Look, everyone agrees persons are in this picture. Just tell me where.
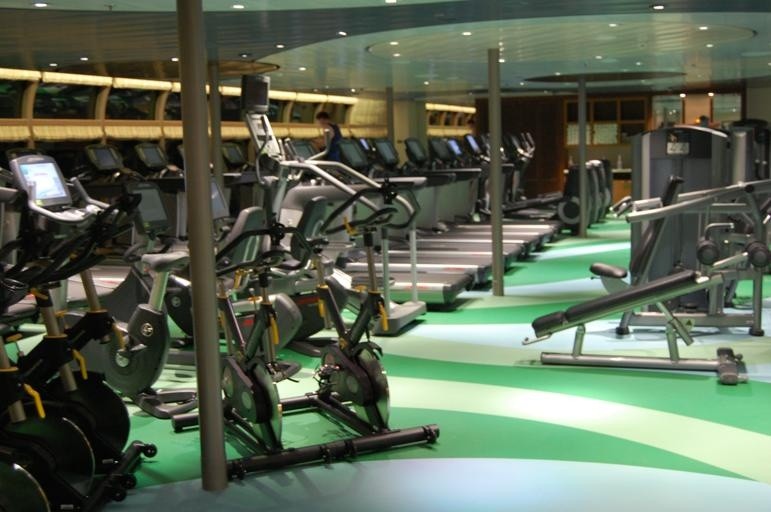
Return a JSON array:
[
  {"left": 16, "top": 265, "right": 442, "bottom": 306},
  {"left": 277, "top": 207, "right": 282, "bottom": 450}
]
[
  {"left": 310, "top": 110, "right": 342, "bottom": 161},
  {"left": 696, "top": 115, "right": 709, "bottom": 128}
]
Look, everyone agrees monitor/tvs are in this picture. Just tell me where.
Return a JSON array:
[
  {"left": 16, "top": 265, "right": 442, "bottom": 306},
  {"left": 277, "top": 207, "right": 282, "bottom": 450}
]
[
  {"left": 288, "top": 134, "right": 482, "bottom": 170},
  {"left": 9, "top": 154, "right": 73, "bottom": 207},
  {"left": 241, "top": 74, "right": 272, "bottom": 113},
  {"left": 221, "top": 142, "right": 247, "bottom": 164},
  {"left": 83, "top": 142, "right": 125, "bottom": 170},
  {"left": 134, "top": 142, "right": 170, "bottom": 168}
]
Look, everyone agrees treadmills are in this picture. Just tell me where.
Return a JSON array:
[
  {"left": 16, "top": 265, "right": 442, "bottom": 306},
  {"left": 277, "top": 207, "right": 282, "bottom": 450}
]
[
  {"left": 351, "top": 135, "right": 526, "bottom": 271},
  {"left": 318, "top": 134, "right": 508, "bottom": 288},
  {"left": 400, "top": 133, "right": 559, "bottom": 271},
  {"left": 284, "top": 137, "right": 476, "bottom": 307}
]
